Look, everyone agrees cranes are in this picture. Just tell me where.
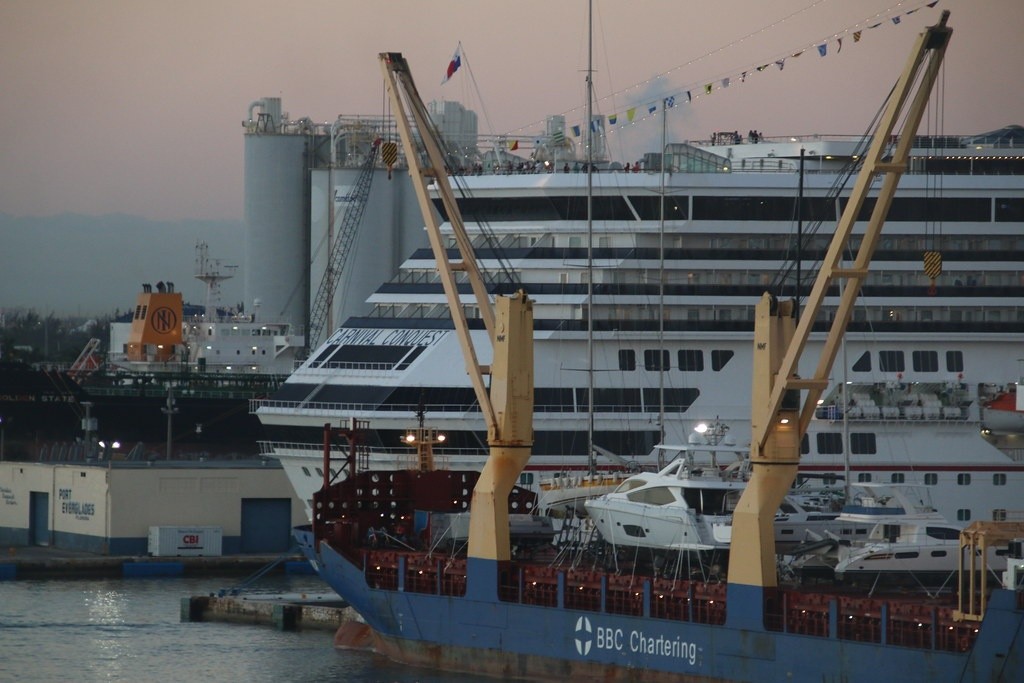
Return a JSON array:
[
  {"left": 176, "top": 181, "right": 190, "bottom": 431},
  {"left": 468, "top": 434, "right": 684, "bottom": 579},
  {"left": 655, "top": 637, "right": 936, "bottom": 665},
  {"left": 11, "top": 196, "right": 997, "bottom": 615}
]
[
  {"left": 724, "top": 10, "right": 955, "bottom": 630},
  {"left": 380, "top": 51, "right": 528, "bottom": 600}
]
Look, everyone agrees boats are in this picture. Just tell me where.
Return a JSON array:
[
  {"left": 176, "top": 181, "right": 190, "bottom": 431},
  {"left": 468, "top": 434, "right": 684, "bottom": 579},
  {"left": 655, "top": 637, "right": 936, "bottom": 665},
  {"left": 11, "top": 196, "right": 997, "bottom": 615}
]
[{"left": 290, "top": 399, "right": 1024, "bottom": 683}]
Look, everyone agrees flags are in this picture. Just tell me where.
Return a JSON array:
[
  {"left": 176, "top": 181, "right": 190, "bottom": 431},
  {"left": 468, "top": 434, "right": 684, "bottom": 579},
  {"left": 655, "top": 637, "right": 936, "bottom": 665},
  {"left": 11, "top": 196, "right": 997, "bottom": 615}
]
[
  {"left": 441, "top": 45, "right": 461, "bottom": 86},
  {"left": 742, "top": 72, "right": 747, "bottom": 81},
  {"left": 705, "top": 84, "right": 712, "bottom": 95},
  {"left": 533, "top": 136, "right": 542, "bottom": 150},
  {"left": 554, "top": 131, "right": 565, "bottom": 145},
  {"left": 509, "top": 140, "right": 520, "bottom": 150},
  {"left": 591, "top": 119, "right": 600, "bottom": 133},
  {"left": 757, "top": 0, "right": 938, "bottom": 72},
  {"left": 722, "top": 79, "right": 729, "bottom": 88},
  {"left": 648, "top": 103, "right": 657, "bottom": 115},
  {"left": 609, "top": 114, "right": 617, "bottom": 125},
  {"left": 627, "top": 107, "right": 634, "bottom": 122},
  {"left": 570, "top": 125, "right": 580, "bottom": 137},
  {"left": 666, "top": 97, "right": 675, "bottom": 109}
]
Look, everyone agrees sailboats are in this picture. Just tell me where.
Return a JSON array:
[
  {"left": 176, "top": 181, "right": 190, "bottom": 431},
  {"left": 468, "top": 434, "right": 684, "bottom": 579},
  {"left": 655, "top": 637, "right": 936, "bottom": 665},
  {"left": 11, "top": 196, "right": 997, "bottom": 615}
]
[{"left": 538, "top": 0, "right": 1009, "bottom": 590}]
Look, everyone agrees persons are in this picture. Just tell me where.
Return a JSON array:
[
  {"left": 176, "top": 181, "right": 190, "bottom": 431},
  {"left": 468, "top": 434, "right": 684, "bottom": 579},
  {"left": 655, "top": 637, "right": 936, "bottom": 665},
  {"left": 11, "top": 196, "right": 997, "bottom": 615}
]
[
  {"left": 445, "top": 159, "right": 639, "bottom": 176},
  {"left": 712, "top": 130, "right": 764, "bottom": 145}
]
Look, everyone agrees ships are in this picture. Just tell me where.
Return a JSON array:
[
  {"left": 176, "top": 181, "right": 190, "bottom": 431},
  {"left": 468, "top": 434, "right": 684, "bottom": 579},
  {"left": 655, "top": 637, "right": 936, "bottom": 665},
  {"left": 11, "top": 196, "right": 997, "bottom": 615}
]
[{"left": 250, "top": 97, "right": 1024, "bottom": 538}]
